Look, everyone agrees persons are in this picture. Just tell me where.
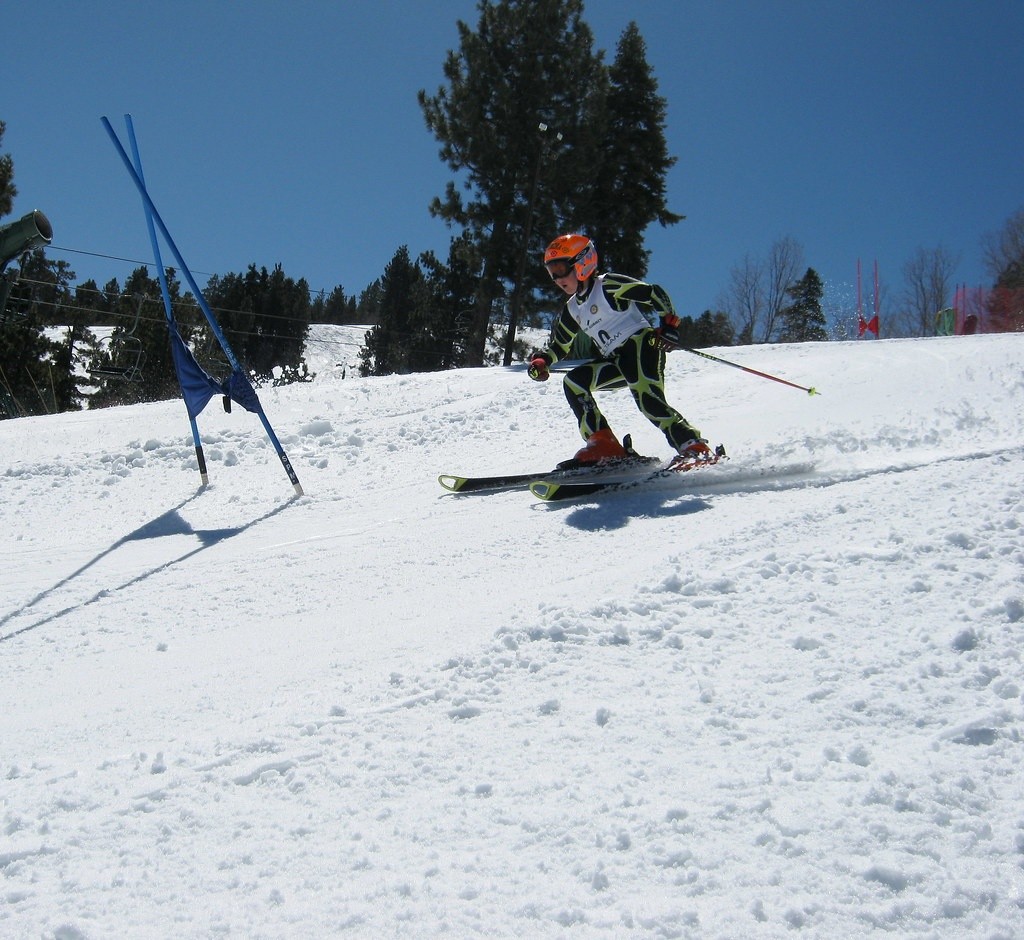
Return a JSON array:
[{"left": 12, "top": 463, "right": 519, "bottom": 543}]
[{"left": 527, "top": 234, "right": 731, "bottom": 482}]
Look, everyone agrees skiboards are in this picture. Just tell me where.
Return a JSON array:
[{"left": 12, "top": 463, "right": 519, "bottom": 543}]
[{"left": 438, "top": 433, "right": 731, "bottom": 503}]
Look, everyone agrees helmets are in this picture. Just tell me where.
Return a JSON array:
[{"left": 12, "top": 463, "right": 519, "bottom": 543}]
[{"left": 543, "top": 234, "right": 598, "bottom": 281}]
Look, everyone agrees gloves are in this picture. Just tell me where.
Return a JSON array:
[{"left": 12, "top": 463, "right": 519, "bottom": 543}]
[
  {"left": 648, "top": 315, "right": 681, "bottom": 353},
  {"left": 528, "top": 352, "right": 553, "bottom": 381}
]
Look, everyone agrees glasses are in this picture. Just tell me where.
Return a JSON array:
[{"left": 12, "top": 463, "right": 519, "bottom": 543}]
[{"left": 543, "top": 256, "right": 574, "bottom": 279}]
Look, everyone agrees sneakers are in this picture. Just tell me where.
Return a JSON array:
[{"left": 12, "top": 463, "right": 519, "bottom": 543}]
[
  {"left": 668, "top": 439, "right": 717, "bottom": 472},
  {"left": 574, "top": 429, "right": 624, "bottom": 461}
]
